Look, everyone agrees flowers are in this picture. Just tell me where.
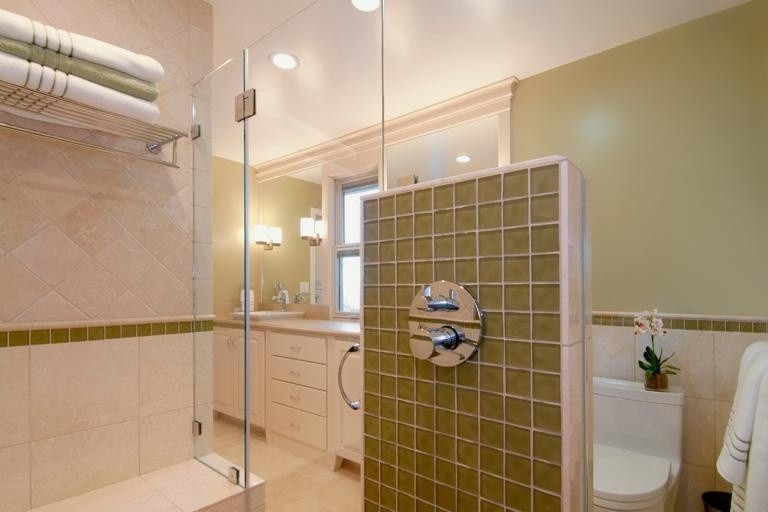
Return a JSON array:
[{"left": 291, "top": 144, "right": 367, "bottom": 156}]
[{"left": 633, "top": 308, "right": 680, "bottom": 380}]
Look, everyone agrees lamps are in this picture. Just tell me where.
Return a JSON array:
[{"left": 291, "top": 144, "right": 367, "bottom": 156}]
[
  {"left": 315, "top": 220, "right": 322, "bottom": 246},
  {"left": 269, "top": 227, "right": 281, "bottom": 250},
  {"left": 255, "top": 226, "right": 269, "bottom": 250},
  {"left": 300, "top": 217, "right": 315, "bottom": 246}
]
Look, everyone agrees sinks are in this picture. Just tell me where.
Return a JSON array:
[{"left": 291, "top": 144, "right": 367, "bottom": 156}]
[{"left": 252, "top": 308, "right": 307, "bottom": 320}]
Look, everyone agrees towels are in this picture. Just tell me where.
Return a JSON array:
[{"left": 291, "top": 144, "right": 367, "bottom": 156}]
[{"left": 0, "top": 9, "right": 162, "bottom": 125}]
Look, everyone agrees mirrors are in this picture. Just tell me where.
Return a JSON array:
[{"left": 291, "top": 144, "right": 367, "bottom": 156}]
[{"left": 258, "top": 162, "right": 323, "bottom": 306}]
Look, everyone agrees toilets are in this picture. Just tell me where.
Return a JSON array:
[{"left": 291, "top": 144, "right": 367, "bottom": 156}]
[{"left": 590, "top": 376, "right": 687, "bottom": 512}]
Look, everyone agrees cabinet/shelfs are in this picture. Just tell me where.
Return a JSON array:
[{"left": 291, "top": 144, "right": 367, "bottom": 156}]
[{"left": 206, "top": 326, "right": 362, "bottom": 479}]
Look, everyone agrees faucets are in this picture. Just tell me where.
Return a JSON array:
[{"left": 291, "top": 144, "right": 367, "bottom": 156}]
[
  {"left": 294, "top": 290, "right": 306, "bottom": 303},
  {"left": 271, "top": 280, "right": 289, "bottom": 312}
]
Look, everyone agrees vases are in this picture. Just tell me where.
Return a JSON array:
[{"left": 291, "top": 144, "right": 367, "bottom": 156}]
[{"left": 643, "top": 373, "right": 668, "bottom": 390}]
